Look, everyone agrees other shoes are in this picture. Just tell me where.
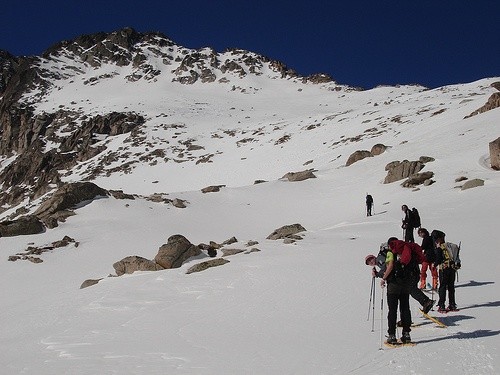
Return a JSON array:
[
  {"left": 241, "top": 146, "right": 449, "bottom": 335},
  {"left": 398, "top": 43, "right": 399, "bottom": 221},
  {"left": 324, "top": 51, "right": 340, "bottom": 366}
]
[
  {"left": 447, "top": 304, "right": 457, "bottom": 310},
  {"left": 438, "top": 305, "right": 445, "bottom": 311},
  {"left": 387, "top": 335, "right": 397, "bottom": 344},
  {"left": 400, "top": 332, "right": 411, "bottom": 343},
  {"left": 397, "top": 321, "right": 403, "bottom": 327},
  {"left": 431, "top": 279, "right": 438, "bottom": 289},
  {"left": 419, "top": 280, "right": 426, "bottom": 289},
  {"left": 423, "top": 300, "right": 436, "bottom": 314}
]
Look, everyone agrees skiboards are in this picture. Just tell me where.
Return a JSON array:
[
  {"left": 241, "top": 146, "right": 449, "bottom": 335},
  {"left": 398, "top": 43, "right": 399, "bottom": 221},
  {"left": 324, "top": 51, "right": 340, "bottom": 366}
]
[
  {"left": 419, "top": 307, "right": 448, "bottom": 328},
  {"left": 383, "top": 339, "right": 417, "bottom": 348},
  {"left": 418, "top": 282, "right": 439, "bottom": 294}
]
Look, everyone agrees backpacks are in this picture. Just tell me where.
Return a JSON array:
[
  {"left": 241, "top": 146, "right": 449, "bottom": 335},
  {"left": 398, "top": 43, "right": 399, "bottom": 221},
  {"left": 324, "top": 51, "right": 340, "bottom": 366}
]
[
  {"left": 445, "top": 242, "right": 461, "bottom": 264},
  {"left": 411, "top": 207, "right": 421, "bottom": 230},
  {"left": 406, "top": 241, "right": 423, "bottom": 264}
]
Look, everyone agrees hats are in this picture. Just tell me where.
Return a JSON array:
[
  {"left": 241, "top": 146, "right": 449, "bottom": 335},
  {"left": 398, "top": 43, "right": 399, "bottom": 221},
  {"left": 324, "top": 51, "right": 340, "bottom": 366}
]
[{"left": 365, "top": 254, "right": 377, "bottom": 266}]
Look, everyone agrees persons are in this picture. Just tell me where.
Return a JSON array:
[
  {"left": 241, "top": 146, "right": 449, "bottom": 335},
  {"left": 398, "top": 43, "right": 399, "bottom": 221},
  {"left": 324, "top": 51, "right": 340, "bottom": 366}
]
[
  {"left": 365, "top": 228, "right": 456, "bottom": 344},
  {"left": 402, "top": 205, "right": 414, "bottom": 242},
  {"left": 366, "top": 195, "right": 373, "bottom": 216}
]
